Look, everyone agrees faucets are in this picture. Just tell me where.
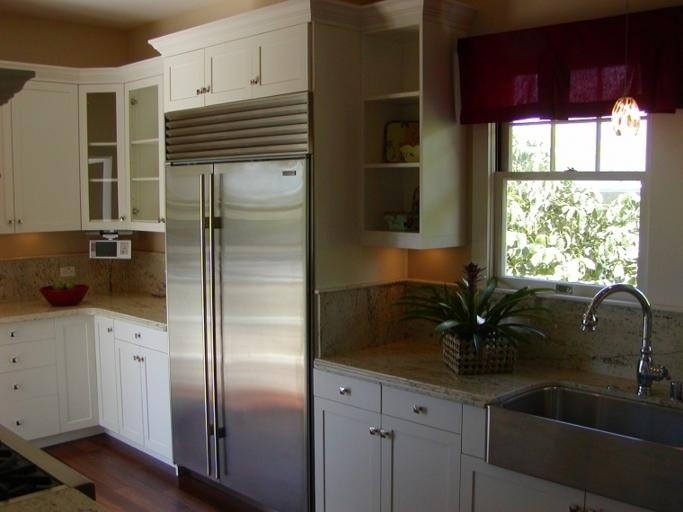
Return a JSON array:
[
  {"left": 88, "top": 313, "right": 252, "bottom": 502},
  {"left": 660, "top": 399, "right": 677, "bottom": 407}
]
[{"left": 581, "top": 284, "right": 670, "bottom": 397}]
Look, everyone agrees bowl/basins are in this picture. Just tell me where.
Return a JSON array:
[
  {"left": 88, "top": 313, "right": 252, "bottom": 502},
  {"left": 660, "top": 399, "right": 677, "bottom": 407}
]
[{"left": 38, "top": 284, "right": 89, "bottom": 307}]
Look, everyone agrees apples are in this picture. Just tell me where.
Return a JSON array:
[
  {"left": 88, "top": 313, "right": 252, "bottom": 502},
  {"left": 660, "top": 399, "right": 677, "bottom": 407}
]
[{"left": 52, "top": 281, "right": 76, "bottom": 291}]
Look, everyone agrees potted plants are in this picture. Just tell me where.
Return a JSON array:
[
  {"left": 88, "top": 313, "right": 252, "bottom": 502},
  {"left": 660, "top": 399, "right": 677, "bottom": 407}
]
[{"left": 391, "top": 259, "right": 560, "bottom": 375}]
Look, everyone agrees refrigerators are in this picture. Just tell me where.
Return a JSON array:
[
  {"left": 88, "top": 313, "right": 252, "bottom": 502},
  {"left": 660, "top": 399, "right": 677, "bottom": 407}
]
[{"left": 166, "top": 159, "right": 309, "bottom": 511}]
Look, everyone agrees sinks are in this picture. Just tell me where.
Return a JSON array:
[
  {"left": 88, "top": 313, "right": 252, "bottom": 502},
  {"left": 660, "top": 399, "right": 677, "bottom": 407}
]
[{"left": 485, "top": 385, "right": 683, "bottom": 508}]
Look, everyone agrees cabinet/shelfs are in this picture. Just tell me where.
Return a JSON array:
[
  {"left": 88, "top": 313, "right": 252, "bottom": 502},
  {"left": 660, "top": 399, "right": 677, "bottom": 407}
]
[
  {"left": 312, "top": 359, "right": 463, "bottom": 512},
  {"left": 0, "top": 307, "right": 99, "bottom": 449},
  {"left": 460, "top": 453, "right": 652, "bottom": 512},
  {"left": 93, "top": 307, "right": 178, "bottom": 475},
  {"left": 0, "top": 55, "right": 166, "bottom": 240},
  {"left": 163, "top": 11, "right": 313, "bottom": 113},
  {"left": 360, "top": 8, "right": 468, "bottom": 251}
]
